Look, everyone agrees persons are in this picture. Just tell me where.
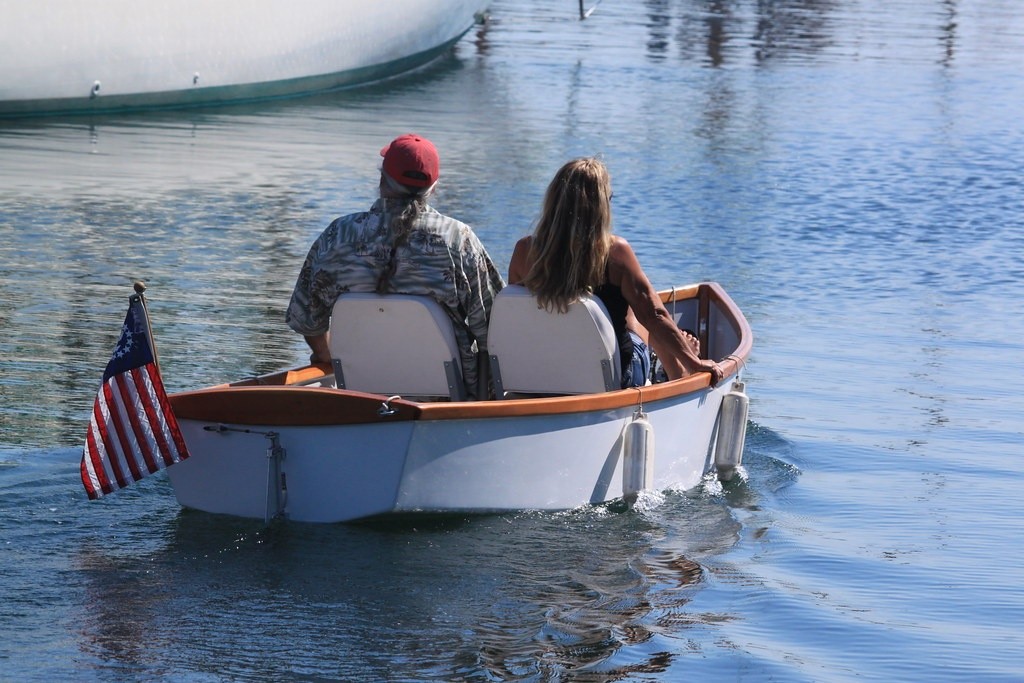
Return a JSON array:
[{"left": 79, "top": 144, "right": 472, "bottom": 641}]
[
  {"left": 286, "top": 133, "right": 508, "bottom": 401},
  {"left": 508, "top": 158, "right": 725, "bottom": 390}
]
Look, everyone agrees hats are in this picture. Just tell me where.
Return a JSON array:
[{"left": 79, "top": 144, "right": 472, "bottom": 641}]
[{"left": 380, "top": 133, "right": 441, "bottom": 187}]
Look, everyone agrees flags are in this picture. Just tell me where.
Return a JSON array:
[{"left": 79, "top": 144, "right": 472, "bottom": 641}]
[{"left": 80, "top": 293, "right": 189, "bottom": 501}]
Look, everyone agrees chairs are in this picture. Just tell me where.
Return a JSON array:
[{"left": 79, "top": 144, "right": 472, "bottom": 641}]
[
  {"left": 327, "top": 292, "right": 468, "bottom": 401},
  {"left": 487, "top": 285, "right": 623, "bottom": 399}
]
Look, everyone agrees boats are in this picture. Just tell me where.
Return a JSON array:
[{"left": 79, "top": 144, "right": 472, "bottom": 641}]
[
  {"left": 158, "top": 278, "right": 755, "bottom": 530},
  {"left": 0, "top": 1, "right": 490, "bottom": 114}
]
